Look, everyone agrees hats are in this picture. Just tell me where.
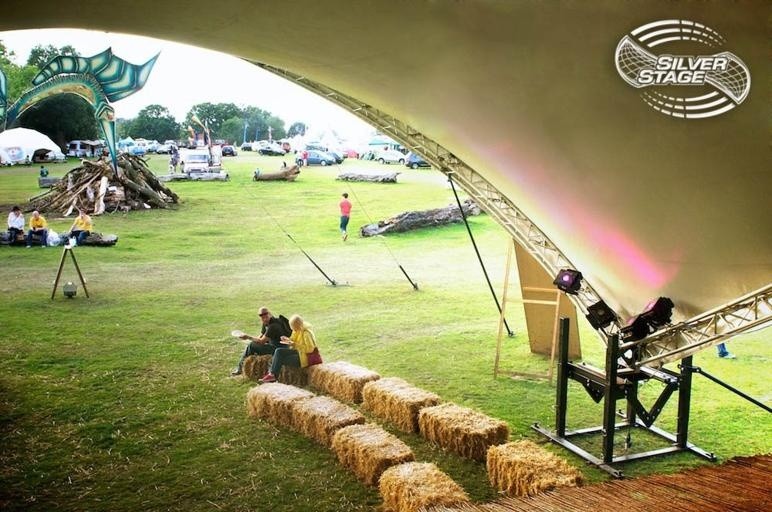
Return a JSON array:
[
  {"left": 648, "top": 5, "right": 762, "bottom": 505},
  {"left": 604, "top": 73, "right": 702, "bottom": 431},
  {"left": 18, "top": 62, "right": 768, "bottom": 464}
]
[{"left": 258, "top": 307, "right": 268, "bottom": 315}]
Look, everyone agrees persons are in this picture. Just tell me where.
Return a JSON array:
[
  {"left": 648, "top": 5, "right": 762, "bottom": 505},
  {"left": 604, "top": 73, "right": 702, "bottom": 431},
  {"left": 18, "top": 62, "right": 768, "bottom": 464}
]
[
  {"left": 24, "top": 155, "right": 32, "bottom": 166},
  {"left": 339, "top": 192, "right": 352, "bottom": 241},
  {"left": 293, "top": 149, "right": 308, "bottom": 167},
  {"left": 168, "top": 145, "right": 185, "bottom": 173},
  {"left": 257, "top": 315, "right": 317, "bottom": 382},
  {"left": 208, "top": 160, "right": 214, "bottom": 173},
  {"left": 68, "top": 209, "right": 92, "bottom": 247},
  {"left": 283, "top": 161, "right": 286, "bottom": 168},
  {"left": 253, "top": 168, "right": 260, "bottom": 181},
  {"left": 232, "top": 307, "right": 293, "bottom": 374},
  {"left": 26, "top": 211, "right": 47, "bottom": 249},
  {"left": 39, "top": 165, "right": 48, "bottom": 177},
  {"left": 8, "top": 206, "right": 24, "bottom": 244}
]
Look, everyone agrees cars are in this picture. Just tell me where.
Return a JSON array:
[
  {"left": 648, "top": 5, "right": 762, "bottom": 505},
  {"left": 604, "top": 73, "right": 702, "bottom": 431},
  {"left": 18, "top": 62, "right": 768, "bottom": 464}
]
[
  {"left": 69, "top": 138, "right": 177, "bottom": 172},
  {"left": 375, "top": 147, "right": 431, "bottom": 169},
  {"left": 241, "top": 140, "right": 359, "bottom": 166},
  {"left": 222, "top": 146, "right": 237, "bottom": 158}
]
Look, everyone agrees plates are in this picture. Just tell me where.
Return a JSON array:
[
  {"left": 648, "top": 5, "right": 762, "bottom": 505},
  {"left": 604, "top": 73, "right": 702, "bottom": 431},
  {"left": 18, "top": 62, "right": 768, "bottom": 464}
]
[
  {"left": 231, "top": 330, "right": 243, "bottom": 338},
  {"left": 279, "top": 340, "right": 293, "bottom": 345}
]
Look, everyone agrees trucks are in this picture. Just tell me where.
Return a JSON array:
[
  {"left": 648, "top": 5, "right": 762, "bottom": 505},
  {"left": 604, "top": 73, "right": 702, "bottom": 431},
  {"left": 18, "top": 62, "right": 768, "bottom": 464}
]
[{"left": 180, "top": 148, "right": 210, "bottom": 173}]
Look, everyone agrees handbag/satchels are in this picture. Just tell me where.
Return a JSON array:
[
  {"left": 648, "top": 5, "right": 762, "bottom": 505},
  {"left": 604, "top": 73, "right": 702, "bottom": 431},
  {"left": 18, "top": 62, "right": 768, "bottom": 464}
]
[{"left": 306, "top": 347, "right": 322, "bottom": 366}]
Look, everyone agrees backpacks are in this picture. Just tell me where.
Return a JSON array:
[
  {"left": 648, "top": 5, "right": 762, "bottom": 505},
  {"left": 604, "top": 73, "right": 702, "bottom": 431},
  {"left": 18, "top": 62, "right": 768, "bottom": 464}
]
[{"left": 265, "top": 315, "right": 292, "bottom": 337}]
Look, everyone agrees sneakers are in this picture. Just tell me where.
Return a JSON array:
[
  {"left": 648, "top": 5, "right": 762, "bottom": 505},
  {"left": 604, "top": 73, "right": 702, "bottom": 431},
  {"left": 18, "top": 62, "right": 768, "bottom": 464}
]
[{"left": 231, "top": 370, "right": 277, "bottom": 385}]
